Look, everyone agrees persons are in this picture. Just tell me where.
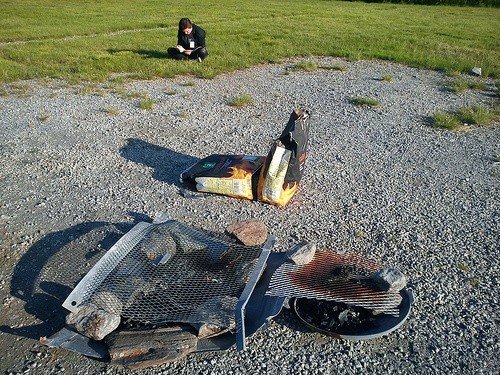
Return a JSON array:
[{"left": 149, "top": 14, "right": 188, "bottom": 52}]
[{"left": 167, "top": 18, "right": 209, "bottom": 62}]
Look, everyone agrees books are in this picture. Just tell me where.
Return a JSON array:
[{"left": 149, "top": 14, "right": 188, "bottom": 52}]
[{"left": 176, "top": 43, "right": 194, "bottom": 53}]
